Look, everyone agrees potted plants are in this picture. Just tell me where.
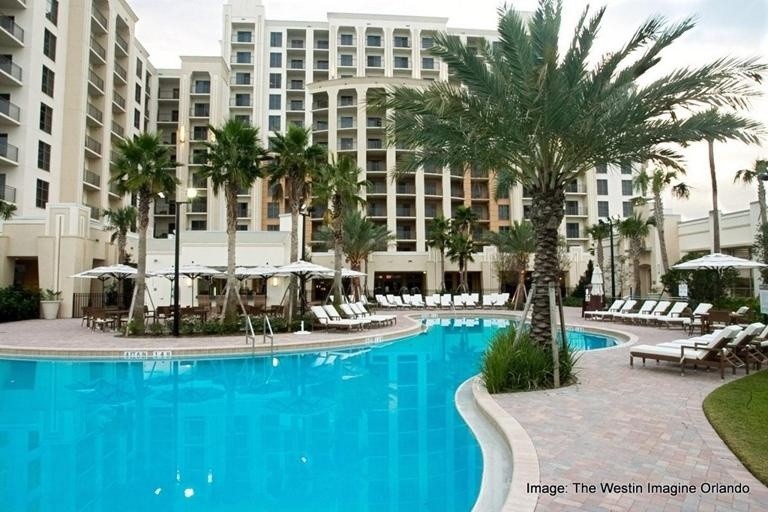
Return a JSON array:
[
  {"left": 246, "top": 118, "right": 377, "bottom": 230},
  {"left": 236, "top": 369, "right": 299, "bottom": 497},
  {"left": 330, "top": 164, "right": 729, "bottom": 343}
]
[{"left": 40, "top": 287, "right": 62, "bottom": 320}]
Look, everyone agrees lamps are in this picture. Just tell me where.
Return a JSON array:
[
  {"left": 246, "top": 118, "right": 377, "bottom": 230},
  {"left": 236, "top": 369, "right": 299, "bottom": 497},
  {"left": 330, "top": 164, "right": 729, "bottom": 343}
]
[
  {"left": 180, "top": 126, "right": 185, "bottom": 143},
  {"left": 211, "top": 132, "right": 216, "bottom": 143}
]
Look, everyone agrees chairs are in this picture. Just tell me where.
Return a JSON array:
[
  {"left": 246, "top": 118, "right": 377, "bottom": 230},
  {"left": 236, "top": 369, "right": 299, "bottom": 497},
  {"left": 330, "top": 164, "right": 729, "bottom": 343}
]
[
  {"left": 80, "top": 295, "right": 288, "bottom": 336},
  {"left": 585, "top": 299, "right": 768, "bottom": 380},
  {"left": 311, "top": 293, "right": 510, "bottom": 334}
]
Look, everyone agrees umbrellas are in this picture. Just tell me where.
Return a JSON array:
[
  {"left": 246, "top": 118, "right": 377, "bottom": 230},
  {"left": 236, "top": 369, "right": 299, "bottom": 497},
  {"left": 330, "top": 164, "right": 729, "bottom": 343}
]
[
  {"left": 672, "top": 252, "right": 768, "bottom": 313},
  {"left": 71, "top": 256, "right": 367, "bottom": 310}
]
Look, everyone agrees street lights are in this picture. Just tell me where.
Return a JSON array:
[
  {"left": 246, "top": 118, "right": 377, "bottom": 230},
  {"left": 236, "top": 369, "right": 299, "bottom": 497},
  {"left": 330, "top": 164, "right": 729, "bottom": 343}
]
[
  {"left": 154, "top": 361, "right": 195, "bottom": 498},
  {"left": 599, "top": 214, "right": 622, "bottom": 304},
  {"left": 299, "top": 203, "right": 316, "bottom": 316},
  {"left": 158, "top": 186, "right": 197, "bottom": 336}
]
